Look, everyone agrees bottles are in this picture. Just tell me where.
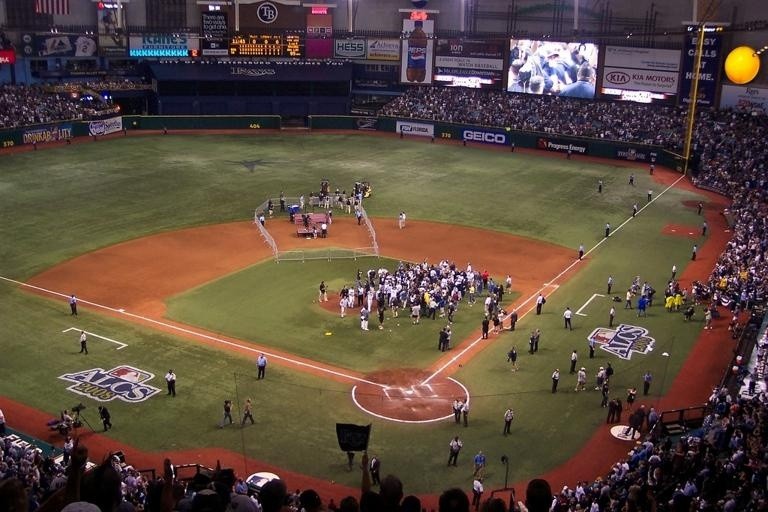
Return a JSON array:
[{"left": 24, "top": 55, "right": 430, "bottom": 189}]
[{"left": 406, "top": 21, "right": 427, "bottom": 83}]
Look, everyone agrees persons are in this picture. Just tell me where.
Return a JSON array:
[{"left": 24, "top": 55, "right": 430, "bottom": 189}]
[
  {"left": 255, "top": 73, "right": 768, "bottom": 511},
  {"left": 2, "top": 74, "right": 166, "bottom": 154},
  {"left": 507, "top": 39, "right": 598, "bottom": 100},
  {"left": 2, "top": 280, "right": 432, "bottom": 511}
]
